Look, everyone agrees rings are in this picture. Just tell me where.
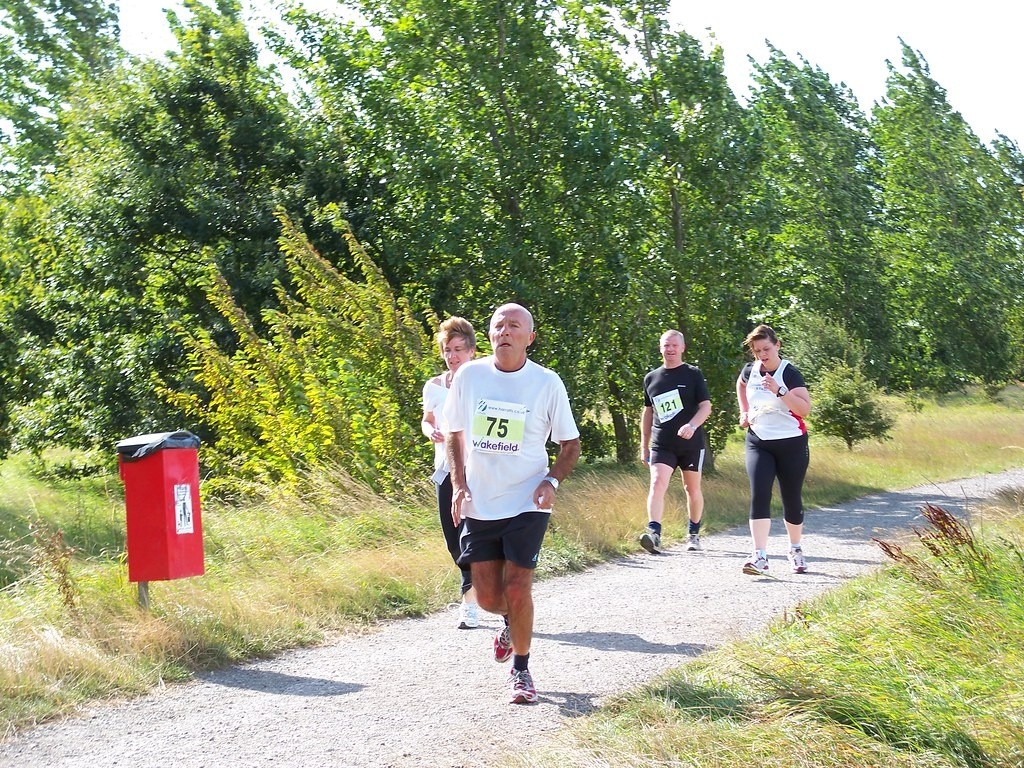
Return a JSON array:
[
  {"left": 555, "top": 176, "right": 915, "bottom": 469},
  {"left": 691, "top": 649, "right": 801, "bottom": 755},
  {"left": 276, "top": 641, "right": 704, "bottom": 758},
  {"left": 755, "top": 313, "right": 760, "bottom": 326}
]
[{"left": 551, "top": 503, "right": 553, "bottom": 506}]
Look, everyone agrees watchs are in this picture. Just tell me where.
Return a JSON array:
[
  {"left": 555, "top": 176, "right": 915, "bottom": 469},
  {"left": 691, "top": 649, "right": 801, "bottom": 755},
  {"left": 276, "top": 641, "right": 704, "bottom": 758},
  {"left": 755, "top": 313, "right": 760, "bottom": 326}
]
[
  {"left": 542, "top": 476, "right": 559, "bottom": 493},
  {"left": 687, "top": 422, "right": 696, "bottom": 431},
  {"left": 776, "top": 386, "right": 786, "bottom": 399}
]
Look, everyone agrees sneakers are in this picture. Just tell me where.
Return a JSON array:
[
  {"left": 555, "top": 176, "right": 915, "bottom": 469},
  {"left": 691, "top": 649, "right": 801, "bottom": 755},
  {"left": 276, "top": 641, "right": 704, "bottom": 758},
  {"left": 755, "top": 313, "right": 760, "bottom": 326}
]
[
  {"left": 493, "top": 625, "right": 514, "bottom": 663},
  {"left": 686, "top": 534, "right": 700, "bottom": 551},
  {"left": 506, "top": 666, "right": 538, "bottom": 703},
  {"left": 639, "top": 527, "right": 661, "bottom": 554},
  {"left": 787, "top": 545, "right": 808, "bottom": 571},
  {"left": 742, "top": 555, "right": 769, "bottom": 576},
  {"left": 457, "top": 601, "right": 478, "bottom": 629}
]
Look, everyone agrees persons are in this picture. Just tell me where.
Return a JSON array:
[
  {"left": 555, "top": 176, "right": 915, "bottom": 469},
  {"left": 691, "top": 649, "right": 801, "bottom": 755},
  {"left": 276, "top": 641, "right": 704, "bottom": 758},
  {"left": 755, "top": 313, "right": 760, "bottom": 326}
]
[
  {"left": 737, "top": 324, "right": 811, "bottom": 576},
  {"left": 640, "top": 330, "right": 712, "bottom": 554},
  {"left": 442, "top": 302, "right": 581, "bottom": 703},
  {"left": 420, "top": 316, "right": 479, "bottom": 629}
]
